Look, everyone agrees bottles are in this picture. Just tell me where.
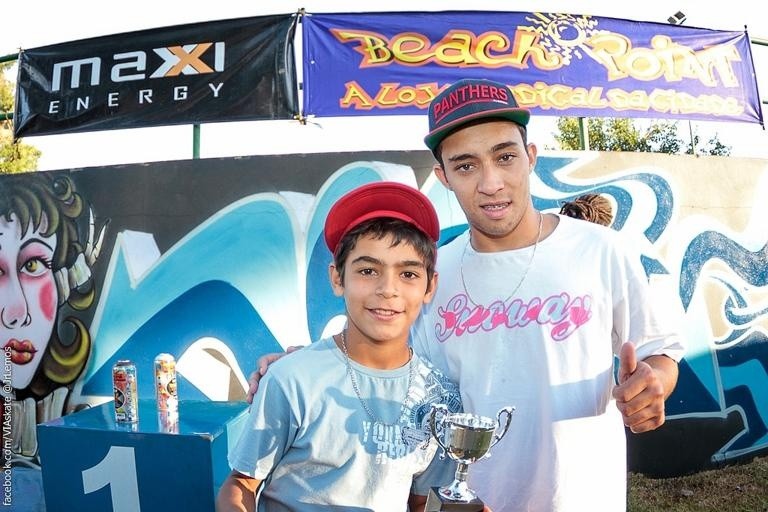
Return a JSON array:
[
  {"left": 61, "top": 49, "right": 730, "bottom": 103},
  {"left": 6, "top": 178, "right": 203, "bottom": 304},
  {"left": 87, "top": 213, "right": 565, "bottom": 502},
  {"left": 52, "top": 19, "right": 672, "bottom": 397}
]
[
  {"left": 421, "top": 76, "right": 530, "bottom": 162},
  {"left": 322, "top": 180, "right": 440, "bottom": 268}
]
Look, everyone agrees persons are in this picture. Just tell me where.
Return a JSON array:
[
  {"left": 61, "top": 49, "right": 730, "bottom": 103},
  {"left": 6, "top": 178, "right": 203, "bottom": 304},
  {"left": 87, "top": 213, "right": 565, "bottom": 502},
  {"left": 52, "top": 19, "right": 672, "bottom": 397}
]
[
  {"left": 245, "top": 78, "right": 687, "bottom": 512},
  {"left": 0, "top": 170, "right": 95, "bottom": 471},
  {"left": 215, "top": 182, "right": 492, "bottom": 512},
  {"left": 559, "top": 194, "right": 613, "bottom": 226}
]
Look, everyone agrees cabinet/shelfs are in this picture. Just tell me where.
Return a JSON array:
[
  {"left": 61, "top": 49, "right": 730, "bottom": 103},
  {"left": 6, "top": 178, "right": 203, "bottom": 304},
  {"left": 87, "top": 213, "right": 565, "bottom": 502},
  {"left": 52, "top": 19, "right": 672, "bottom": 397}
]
[
  {"left": 111, "top": 359, "right": 139, "bottom": 424},
  {"left": 153, "top": 352, "right": 179, "bottom": 412},
  {"left": 157, "top": 411, "right": 179, "bottom": 435}
]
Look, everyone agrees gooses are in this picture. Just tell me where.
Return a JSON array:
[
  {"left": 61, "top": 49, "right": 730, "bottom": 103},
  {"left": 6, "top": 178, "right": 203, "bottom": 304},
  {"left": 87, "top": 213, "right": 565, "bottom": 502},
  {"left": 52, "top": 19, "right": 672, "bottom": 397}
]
[{"left": 422, "top": 402, "right": 515, "bottom": 507}]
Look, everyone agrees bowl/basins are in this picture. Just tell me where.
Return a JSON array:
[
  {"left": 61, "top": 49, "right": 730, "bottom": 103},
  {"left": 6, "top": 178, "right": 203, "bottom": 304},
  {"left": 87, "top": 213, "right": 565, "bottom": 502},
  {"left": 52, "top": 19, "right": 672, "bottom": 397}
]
[
  {"left": 341, "top": 329, "right": 413, "bottom": 426},
  {"left": 460, "top": 210, "right": 543, "bottom": 310}
]
